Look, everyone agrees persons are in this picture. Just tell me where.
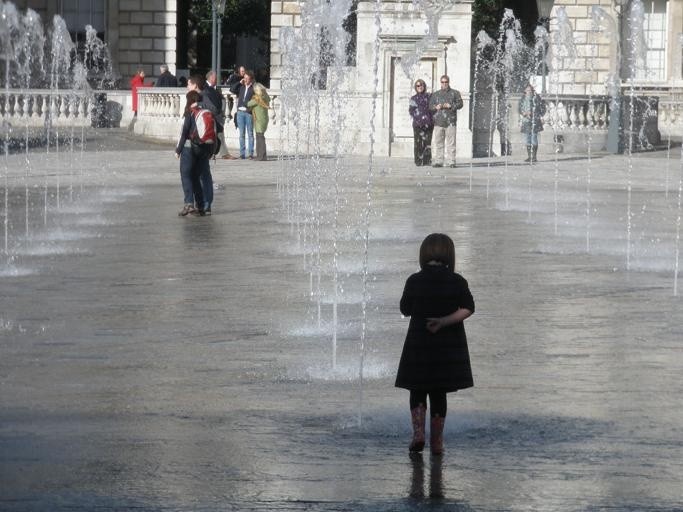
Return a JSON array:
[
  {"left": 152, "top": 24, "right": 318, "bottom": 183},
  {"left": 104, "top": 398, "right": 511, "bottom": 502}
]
[
  {"left": 429, "top": 76, "right": 463, "bottom": 169},
  {"left": 517, "top": 85, "right": 547, "bottom": 163},
  {"left": 130, "top": 64, "right": 272, "bottom": 219},
  {"left": 408, "top": 79, "right": 434, "bottom": 166},
  {"left": 395, "top": 233, "right": 474, "bottom": 456}
]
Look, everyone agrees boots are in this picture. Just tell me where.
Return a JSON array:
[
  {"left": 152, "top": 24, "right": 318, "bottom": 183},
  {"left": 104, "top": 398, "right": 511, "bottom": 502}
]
[
  {"left": 409, "top": 403, "right": 426, "bottom": 452},
  {"left": 429, "top": 413, "right": 444, "bottom": 455}
]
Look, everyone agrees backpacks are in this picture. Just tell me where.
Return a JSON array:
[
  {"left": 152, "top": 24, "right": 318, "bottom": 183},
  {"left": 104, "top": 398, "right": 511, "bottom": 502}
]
[{"left": 186, "top": 106, "right": 216, "bottom": 146}]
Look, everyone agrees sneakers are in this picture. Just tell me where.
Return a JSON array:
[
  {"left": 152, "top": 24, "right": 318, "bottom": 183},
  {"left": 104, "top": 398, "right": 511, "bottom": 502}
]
[{"left": 179, "top": 203, "right": 212, "bottom": 217}]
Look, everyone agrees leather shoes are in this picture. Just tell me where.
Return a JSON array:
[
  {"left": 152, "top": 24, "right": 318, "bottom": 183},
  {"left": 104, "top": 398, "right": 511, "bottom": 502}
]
[{"left": 222, "top": 154, "right": 253, "bottom": 160}]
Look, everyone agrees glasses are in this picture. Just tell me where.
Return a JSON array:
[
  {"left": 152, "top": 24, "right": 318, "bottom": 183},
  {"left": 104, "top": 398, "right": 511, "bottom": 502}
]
[{"left": 441, "top": 81, "right": 447, "bottom": 83}]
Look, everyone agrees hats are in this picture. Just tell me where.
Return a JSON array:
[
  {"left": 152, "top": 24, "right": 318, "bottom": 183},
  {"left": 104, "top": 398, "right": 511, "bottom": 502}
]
[{"left": 252, "top": 82, "right": 266, "bottom": 97}]
